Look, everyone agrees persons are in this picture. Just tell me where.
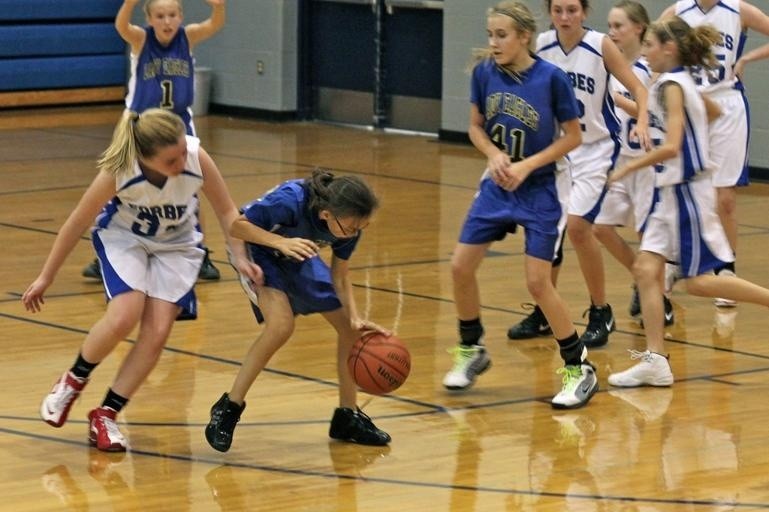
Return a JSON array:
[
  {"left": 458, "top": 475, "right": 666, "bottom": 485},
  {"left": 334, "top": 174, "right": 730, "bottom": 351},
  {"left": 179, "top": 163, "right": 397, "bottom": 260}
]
[
  {"left": 442, "top": 0, "right": 597, "bottom": 410},
  {"left": 21, "top": 108, "right": 264, "bottom": 452},
  {"left": 206, "top": 170, "right": 391, "bottom": 452},
  {"left": 658, "top": 0, "right": 769, "bottom": 307},
  {"left": 606, "top": 16, "right": 769, "bottom": 387},
  {"left": 507, "top": 0, "right": 653, "bottom": 348},
  {"left": 591, "top": 0, "right": 673, "bottom": 328},
  {"left": 82, "top": 0, "right": 225, "bottom": 284}
]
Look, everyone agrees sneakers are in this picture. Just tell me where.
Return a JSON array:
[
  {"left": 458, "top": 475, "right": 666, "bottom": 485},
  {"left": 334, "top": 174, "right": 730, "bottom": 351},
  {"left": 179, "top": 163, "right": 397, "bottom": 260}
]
[
  {"left": 713, "top": 268, "right": 737, "bottom": 306},
  {"left": 628, "top": 284, "right": 674, "bottom": 329},
  {"left": 87, "top": 404, "right": 127, "bottom": 452},
  {"left": 441, "top": 338, "right": 491, "bottom": 391},
  {"left": 579, "top": 303, "right": 615, "bottom": 347},
  {"left": 608, "top": 349, "right": 673, "bottom": 387},
  {"left": 329, "top": 405, "right": 391, "bottom": 446},
  {"left": 550, "top": 358, "right": 599, "bottom": 409},
  {"left": 507, "top": 303, "right": 553, "bottom": 339},
  {"left": 198, "top": 244, "right": 220, "bottom": 280},
  {"left": 205, "top": 392, "right": 246, "bottom": 452},
  {"left": 81, "top": 254, "right": 102, "bottom": 279},
  {"left": 53, "top": 404, "right": 56, "bottom": 406},
  {"left": 40, "top": 368, "right": 91, "bottom": 428}
]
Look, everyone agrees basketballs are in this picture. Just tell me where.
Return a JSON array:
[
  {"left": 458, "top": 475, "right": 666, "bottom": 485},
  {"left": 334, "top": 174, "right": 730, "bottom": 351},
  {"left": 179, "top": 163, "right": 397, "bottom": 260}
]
[{"left": 347, "top": 331, "right": 411, "bottom": 394}]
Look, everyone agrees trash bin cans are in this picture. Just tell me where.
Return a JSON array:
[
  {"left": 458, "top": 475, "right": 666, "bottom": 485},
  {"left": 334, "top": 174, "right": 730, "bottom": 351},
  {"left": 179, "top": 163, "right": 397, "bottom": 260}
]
[{"left": 189, "top": 67, "right": 212, "bottom": 116}]
